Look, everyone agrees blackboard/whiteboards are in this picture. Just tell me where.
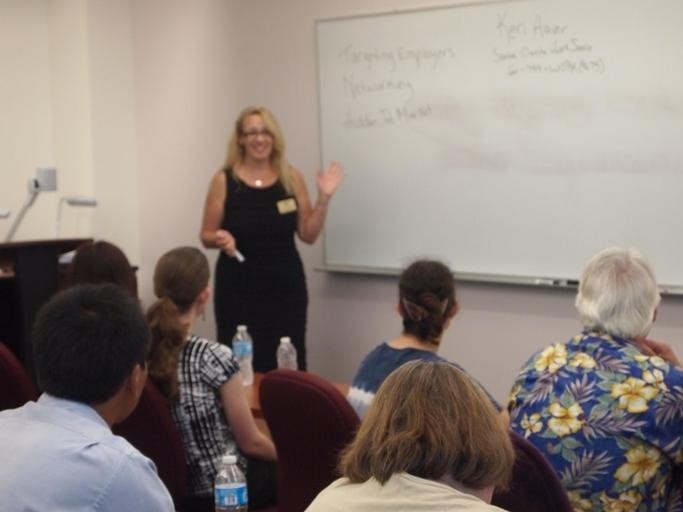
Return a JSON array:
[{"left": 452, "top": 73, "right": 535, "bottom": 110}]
[{"left": 315, "top": 0, "right": 682, "bottom": 296}]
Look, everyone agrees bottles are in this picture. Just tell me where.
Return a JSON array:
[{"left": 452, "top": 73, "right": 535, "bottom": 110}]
[
  {"left": 276, "top": 336, "right": 298, "bottom": 374},
  {"left": 213, "top": 456, "right": 249, "bottom": 510},
  {"left": 231, "top": 324, "right": 254, "bottom": 387}
]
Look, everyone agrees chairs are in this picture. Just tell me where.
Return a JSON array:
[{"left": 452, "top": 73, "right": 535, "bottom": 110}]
[
  {"left": 491, "top": 432, "right": 574, "bottom": 512},
  {"left": 258, "top": 370, "right": 364, "bottom": 512},
  {"left": 2, "top": 342, "right": 37, "bottom": 410},
  {"left": 112, "top": 376, "right": 200, "bottom": 512}
]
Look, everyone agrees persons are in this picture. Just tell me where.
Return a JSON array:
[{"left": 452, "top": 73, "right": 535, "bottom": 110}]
[
  {"left": 144, "top": 247, "right": 277, "bottom": 498},
  {"left": 1, "top": 283, "right": 180, "bottom": 512},
  {"left": 505, "top": 245, "right": 682, "bottom": 510},
  {"left": 346, "top": 260, "right": 510, "bottom": 425},
  {"left": 201, "top": 102, "right": 349, "bottom": 374},
  {"left": 304, "top": 360, "right": 515, "bottom": 512}
]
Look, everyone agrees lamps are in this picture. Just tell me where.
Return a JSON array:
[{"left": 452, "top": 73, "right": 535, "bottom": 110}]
[{"left": 1, "top": 162, "right": 98, "bottom": 241}]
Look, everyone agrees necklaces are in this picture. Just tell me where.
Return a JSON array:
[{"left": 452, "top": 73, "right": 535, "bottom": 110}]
[{"left": 237, "top": 159, "right": 278, "bottom": 187}]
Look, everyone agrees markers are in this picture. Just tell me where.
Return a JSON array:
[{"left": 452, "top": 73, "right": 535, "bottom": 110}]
[
  {"left": 235, "top": 251, "right": 246, "bottom": 263},
  {"left": 534, "top": 279, "right": 558, "bottom": 285},
  {"left": 560, "top": 280, "right": 580, "bottom": 285}
]
